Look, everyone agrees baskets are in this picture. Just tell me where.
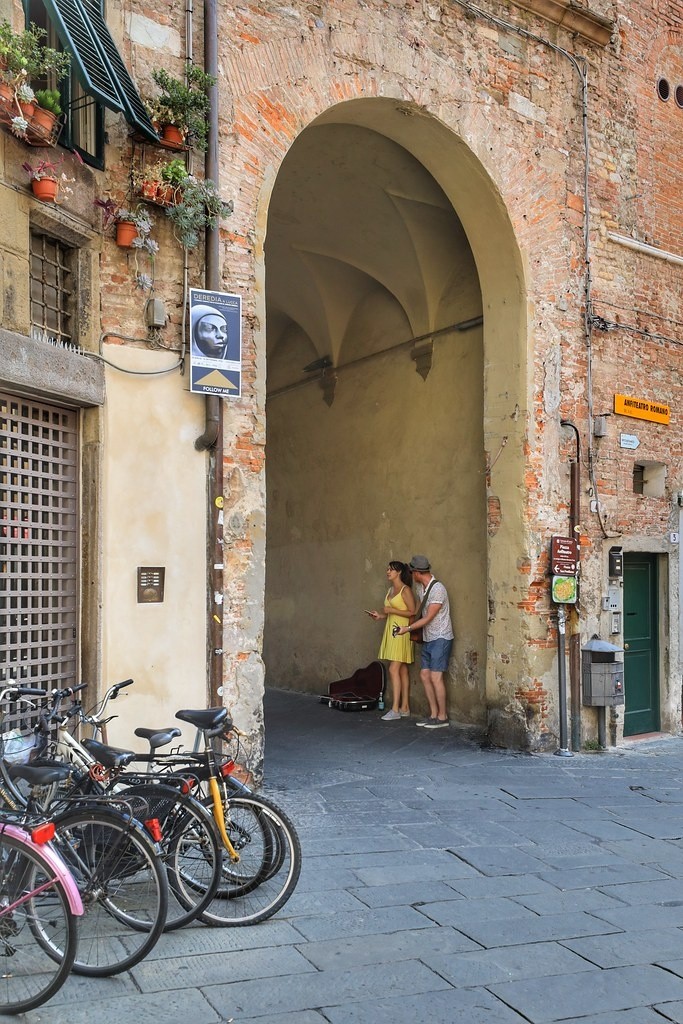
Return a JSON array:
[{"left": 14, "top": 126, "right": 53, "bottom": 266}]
[{"left": 3, "top": 720, "right": 45, "bottom": 765}]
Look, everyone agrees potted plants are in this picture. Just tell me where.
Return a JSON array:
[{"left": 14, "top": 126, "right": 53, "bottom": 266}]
[{"left": 150, "top": 60, "right": 212, "bottom": 151}]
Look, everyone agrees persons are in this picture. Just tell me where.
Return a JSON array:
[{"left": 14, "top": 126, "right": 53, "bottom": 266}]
[
  {"left": 393, "top": 555, "right": 455, "bottom": 729},
  {"left": 366, "top": 560, "right": 416, "bottom": 721}
]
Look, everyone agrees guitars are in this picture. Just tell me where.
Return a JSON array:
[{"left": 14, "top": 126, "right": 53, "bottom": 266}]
[{"left": 393, "top": 616, "right": 423, "bottom": 642}]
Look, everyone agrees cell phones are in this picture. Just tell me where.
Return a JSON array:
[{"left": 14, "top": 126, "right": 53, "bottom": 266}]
[{"left": 365, "top": 610, "right": 377, "bottom": 616}]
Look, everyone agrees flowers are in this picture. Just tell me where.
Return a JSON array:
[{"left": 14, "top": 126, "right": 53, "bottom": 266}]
[{"left": 0, "top": 15, "right": 232, "bottom": 286}]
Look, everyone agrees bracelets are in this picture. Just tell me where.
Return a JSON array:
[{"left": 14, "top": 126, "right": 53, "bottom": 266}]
[{"left": 407, "top": 626, "right": 414, "bottom": 633}]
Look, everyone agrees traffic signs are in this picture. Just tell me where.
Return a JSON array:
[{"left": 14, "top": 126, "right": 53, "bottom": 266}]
[{"left": 551, "top": 536, "right": 580, "bottom": 575}]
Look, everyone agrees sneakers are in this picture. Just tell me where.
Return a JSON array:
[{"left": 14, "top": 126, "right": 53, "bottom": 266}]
[
  {"left": 424, "top": 719, "right": 449, "bottom": 729},
  {"left": 416, "top": 717, "right": 432, "bottom": 727},
  {"left": 381, "top": 711, "right": 401, "bottom": 721},
  {"left": 399, "top": 709, "right": 410, "bottom": 716}
]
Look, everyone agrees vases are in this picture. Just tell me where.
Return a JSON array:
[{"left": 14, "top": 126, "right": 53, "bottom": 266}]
[
  {"left": 157, "top": 182, "right": 172, "bottom": 200},
  {"left": 31, "top": 177, "right": 58, "bottom": 202},
  {"left": 142, "top": 179, "right": 159, "bottom": 196},
  {"left": 13, "top": 95, "right": 38, "bottom": 116},
  {"left": 1, "top": 83, "right": 14, "bottom": 113},
  {"left": 31, "top": 108, "right": 57, "bottom": 139},
  {"left": 116, "top": 220, "right": 137, "bottom": 249}
]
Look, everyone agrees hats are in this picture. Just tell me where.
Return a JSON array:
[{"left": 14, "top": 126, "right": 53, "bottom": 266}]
[{"left": 409, "top": 555, "right": 432, "bottom": 572}]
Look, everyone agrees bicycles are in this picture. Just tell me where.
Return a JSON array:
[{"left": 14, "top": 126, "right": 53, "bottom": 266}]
[
  {"left": 0, "top": 679, "right": 302, "bottom": 979},
  {"left": 0, "top": 802, "right": 85, "bottom": 1016}
]
